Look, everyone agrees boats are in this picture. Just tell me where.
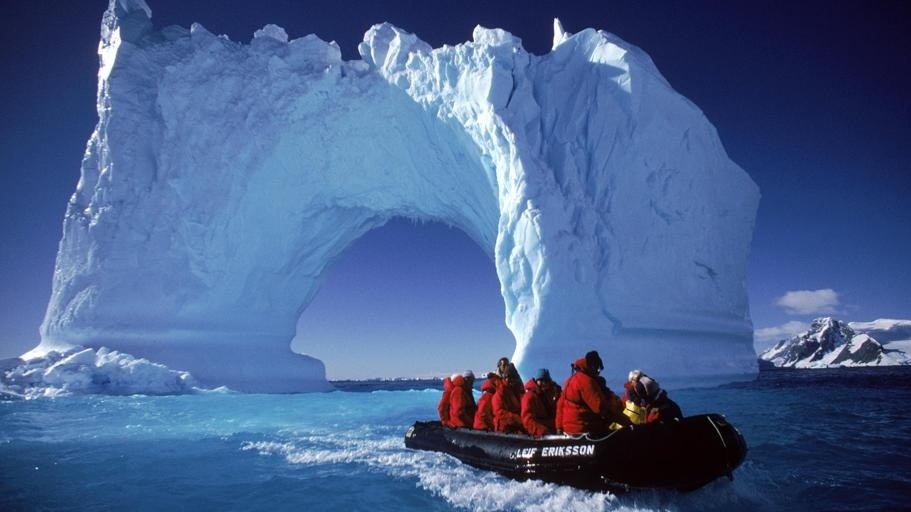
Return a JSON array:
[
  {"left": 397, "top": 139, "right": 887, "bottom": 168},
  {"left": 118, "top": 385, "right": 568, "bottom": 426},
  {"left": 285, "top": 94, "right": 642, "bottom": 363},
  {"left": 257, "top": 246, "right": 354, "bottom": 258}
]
[{"left": 400, "top": 411, "right": 748, "bottom": 506}]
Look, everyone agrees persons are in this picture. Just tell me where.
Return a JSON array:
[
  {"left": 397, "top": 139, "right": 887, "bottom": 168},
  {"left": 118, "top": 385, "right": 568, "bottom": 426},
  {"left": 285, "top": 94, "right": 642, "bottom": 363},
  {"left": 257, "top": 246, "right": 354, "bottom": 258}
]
[{"left": 438, "top": 350, "right": 685, "bottom": 436}]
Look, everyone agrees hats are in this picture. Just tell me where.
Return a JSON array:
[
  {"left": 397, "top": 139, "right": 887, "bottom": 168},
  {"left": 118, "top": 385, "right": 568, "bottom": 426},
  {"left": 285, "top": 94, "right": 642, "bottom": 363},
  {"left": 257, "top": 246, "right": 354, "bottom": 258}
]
[
  {"left": 585, "top": 351, "right": 605, "bottom": 372},
  {"left": 534, "top": 367, "right": 553, "bottom": 382},
  {"left": 451, "top": 369, "right": 475, "bottom": 381}
]
